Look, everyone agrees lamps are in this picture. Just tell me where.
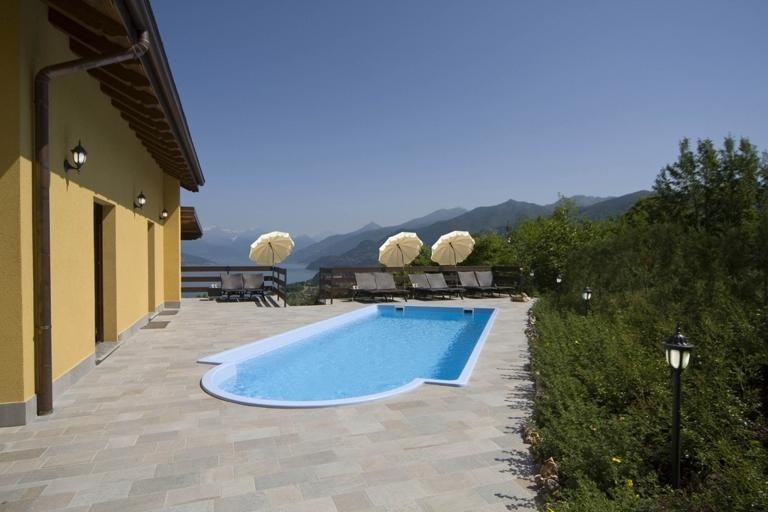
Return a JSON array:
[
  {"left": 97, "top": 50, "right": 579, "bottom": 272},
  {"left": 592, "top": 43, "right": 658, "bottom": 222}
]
[
  {"left": 134, "top": 190, "right": 146, "bottom": 209},
  {"left": 159, "top": 208, "right": 168, "bottom": 220},
  {"left": 63, "top": 138, "right": 87, "bottom": 175}
]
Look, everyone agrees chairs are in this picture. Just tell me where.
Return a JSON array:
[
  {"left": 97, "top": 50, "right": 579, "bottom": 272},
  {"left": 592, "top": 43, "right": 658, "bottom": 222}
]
[
  {"left": 220, "top": 273, "right": 244, "bottom": 302},
  {"left": 353, "top": 270, "right": 517, "bottom": 301},
  {"left": 243, "top": 272, "right": 265, "bottom": 302}
]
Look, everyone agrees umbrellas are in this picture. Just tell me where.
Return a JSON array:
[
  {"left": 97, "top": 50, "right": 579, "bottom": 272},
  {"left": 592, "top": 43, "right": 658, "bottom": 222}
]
[
  {"left": 430, "top": 231, "right": 475, "bottom": 288},
  {"left": 379, "top": 232, "right": 424, "bottom": 290},
  {"left": 249, "top": 231, "right": 295, "bottom": 285}
]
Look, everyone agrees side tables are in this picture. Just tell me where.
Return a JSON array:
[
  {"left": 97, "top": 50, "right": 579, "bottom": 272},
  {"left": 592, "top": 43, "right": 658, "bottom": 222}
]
[{"left": 208, "top": 287, "right": 221, "bottom": 303}]
